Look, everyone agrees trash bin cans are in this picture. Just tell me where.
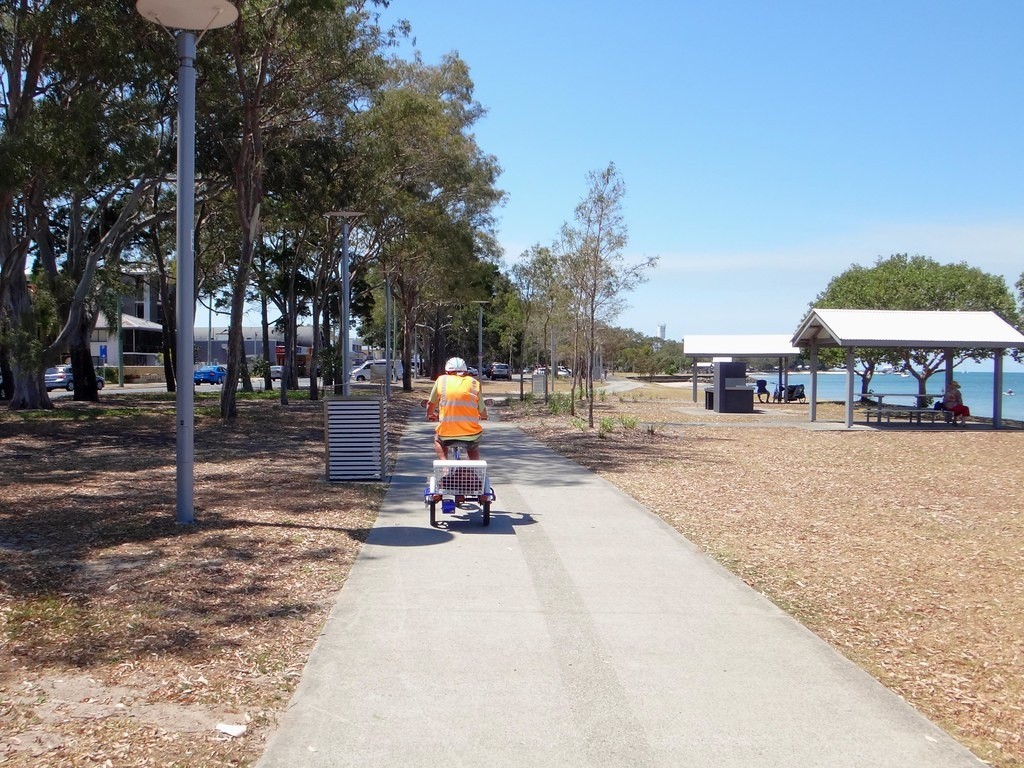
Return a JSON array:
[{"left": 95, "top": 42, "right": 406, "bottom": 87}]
[
  {"left": 531, "top": 374, "right": 546, "bottom": 394},
  {"left": 321, "top": 397, "right": 388, "bottom": 480}
]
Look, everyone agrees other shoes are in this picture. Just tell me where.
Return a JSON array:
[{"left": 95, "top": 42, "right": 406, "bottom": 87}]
[{"left": 442, "top": 467, "right": 449, "bottom": 478}]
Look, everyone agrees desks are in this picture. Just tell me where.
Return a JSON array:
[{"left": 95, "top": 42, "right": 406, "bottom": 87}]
[{"left": 853, "top": 393, "right": 945, "bottom": 425}]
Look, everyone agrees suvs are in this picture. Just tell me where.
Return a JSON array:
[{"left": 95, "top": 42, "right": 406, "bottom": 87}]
[{"left": 491, "top": 364, "right": 513, "bottom": 381}]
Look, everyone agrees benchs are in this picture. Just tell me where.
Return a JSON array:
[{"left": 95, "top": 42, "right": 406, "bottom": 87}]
[
  {"left": 860, "top": 409, "right": 957, "bottom": 425},
  {"left": 753, "top": 392, "right": 767, "bottom": 395}
]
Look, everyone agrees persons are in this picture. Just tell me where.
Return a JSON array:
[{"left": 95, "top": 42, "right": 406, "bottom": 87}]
[
  {"left": 757, "top": 379, "right": 770, "bottom": 403},
  {"left": 943, "top": 381, "right": 971, "bottom": 428},
  {"left": 425, "top": 358, "right": 488, "bottom": 479}
]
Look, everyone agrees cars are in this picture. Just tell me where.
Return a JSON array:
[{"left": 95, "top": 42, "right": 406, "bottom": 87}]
[
  {"left": 271, "top": 365, "right": 285, "bottom": 381},
  {"left": 465, "top": 363, "right": 491, "bottom": 379},
  {"left": 43, "top": 365, "right": 106, "bottom": 392},
  {"left": 410, "top": 358, "right": 425, "bottom": 373},
  {"left": 194, "top": 365, "right": 226, "bottom": 386},
  {"left": 523, "top": 362, "right": 572, "bottom": 378}
]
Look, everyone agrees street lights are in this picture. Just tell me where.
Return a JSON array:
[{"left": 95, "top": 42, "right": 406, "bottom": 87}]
[{"left": 469, "top": 300, "right": 490, "bottom": 393}]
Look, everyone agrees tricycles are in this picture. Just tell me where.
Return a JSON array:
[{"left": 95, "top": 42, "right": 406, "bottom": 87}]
[
  {"left": 770, "top": 381, "right": 807, "bottom": 404},
  {"left": 420, "top": 397, "right": 497, "bottom": 527}
]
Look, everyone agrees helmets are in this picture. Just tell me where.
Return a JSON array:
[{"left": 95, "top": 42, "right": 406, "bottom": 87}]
[{"left": 445, "top": 357, "right": 467, "bottom": 372}]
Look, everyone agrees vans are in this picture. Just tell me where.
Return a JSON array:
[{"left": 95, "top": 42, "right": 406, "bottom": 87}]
[{"left": 351, "top": 359, "right": 403, "bottom": 382}]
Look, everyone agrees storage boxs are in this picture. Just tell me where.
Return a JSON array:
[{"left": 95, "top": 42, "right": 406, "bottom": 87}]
[{"left": 432, "top": 460, "right": 487, "bottom": 496}]
